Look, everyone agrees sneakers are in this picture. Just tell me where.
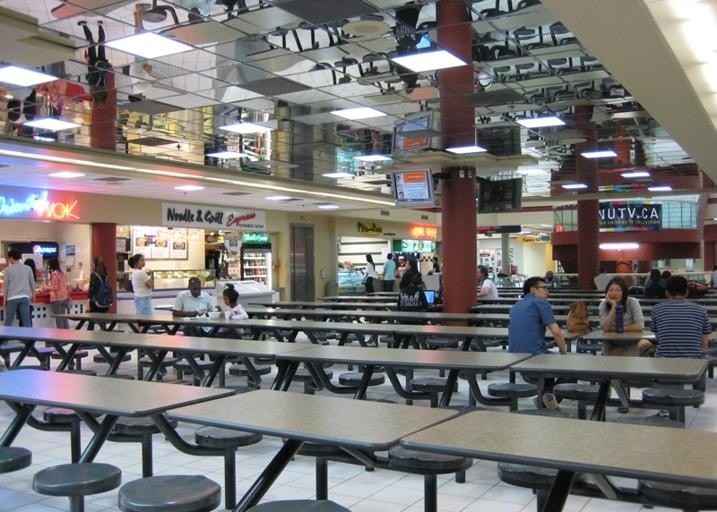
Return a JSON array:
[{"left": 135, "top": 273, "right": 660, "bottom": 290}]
[
  {"left": 543, "top": 392, "right": 559, "bottom": 411},
  {"left": 533, "top": 396, "right": 546, "bottom": 410}
]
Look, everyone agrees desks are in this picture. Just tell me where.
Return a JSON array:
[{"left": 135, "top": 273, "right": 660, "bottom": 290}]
[
  {"left": 0, "top": 368, "right": 237, "bottom": 511},
  {"left": 0, "top": 326, "right": 119, "bottom": 370},
  {"left": 107, "top": 335, "right": 321, "bottom": 388},
  {"left": 275, "top": 345, "right": 533, "bottom": 408},
  {"left": 164, "top": 388, "right": 460, "bottom": 511},
  {"left": 510, "top": 353, "right": 710, "bottom": 422},
  {"left": 400, "top": 409, "right": 717, "bottom": 511},
  {"left": 50, "top": 285, "right": 716, "bottom": 349}
]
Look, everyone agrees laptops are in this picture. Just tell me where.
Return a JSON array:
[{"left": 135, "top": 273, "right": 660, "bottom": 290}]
[{"left": 423, "top": 289, "right": 436, "bottom": 306}]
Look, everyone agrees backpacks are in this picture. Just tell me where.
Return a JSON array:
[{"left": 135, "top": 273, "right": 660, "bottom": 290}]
[{"left": 92, "top": 272, "right": 113, "bottom": 308}]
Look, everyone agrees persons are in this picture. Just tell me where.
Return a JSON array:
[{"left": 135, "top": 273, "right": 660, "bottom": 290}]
[
  {"left": 363, "top": 254, "right": 377, "bottom": 293},
  {"left": 660, "top": 271, "right": 674, "bottom": 288},
  {"left": 386, "top": 6, "right": 427, "bottom": 94},
  {"left": 87, "top": 255, "right": 113, "bottom": 313},
  {"left": 509, "top": 277, "right": 565, "bottom": 339},
  {"left": 399, "top": 258, "right": 419, "bottom": 290},
  {"left": 171, "top": 276, "right": 215, "bottom": 335},
  {"left": 214, "top": 281, "right": 247, "bottom": 338},
  {"left": 598, "top": 277, "right": 645, "bottom": 332},
  {"left": 476, "top": 265, "right": 499, "bottom": 301},
  {"left": 1, "top": 249, "right": 36, "bottom": 346},
  {"left": 42, "top": 257, "right": 69, "bottom": 329},
  {"left": 638, "top": 275, "right": 712, "bottom": 358},
  {"left": 382, "top": 253, "right": 396, "bottom": 292},
  {"left": 430, "top": 256, "right": 440, "bottom": 274},
  {"left": 128, "top": 254, "right": 155, "bottom": 314},
  {"left": 398, "top": 273, "right": 429, "bottom": 312},
  {"left": 77, "top": 16, "right": 112, "bottom": 103},
  {"left": 644, "top": 269, "right": 666, "bottom": 299}
]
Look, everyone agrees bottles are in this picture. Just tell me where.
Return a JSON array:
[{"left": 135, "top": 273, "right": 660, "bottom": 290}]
[{"left": 615, "top": 305, "right": 624, "bottom": 334}]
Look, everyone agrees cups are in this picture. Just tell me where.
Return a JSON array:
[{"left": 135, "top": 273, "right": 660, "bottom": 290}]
[{"left": 225, "top": 311, "right": 230, "bottom": 320}]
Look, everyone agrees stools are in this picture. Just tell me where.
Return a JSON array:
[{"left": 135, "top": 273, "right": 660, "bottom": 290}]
[
  {"left": 282, "top": 438, "right": 340, "bottom": 500},
  {"left": 194, "top": 424, "right": 263, "bottom": 509},
  {"left": 293, "top": 367, "right": 333, "bottom": 395},
  {"left": 140, "top": 357, "right": 179, "bottom": 381},
  {"left": 339, "top": 373, "right": 385, "bottom": 401},
  {"left": 173, "top": 360, "right": 215, "bottom": 388},
  {"left": 643, "top": 388, "right": 705, "bottom": 426},
  {"left": 27, "top": 347, "right": 56, "bottom": 370},
  {"left": 495, "top": 461, "right": 559, "bottom": 511},
  {"left": 44, "top": 407, "right": 104, "bottom": 464},
  {"left": 410, "top": 378, "right": 455, "bottom": 406},
  {"left": 32, "top": 462, "right": 122, "bottom": 512},
  {"left": 388, "top": 446, "right": 472, "bottom": 510},
  {"left": 105, "top": 374, "right": 135, "bottom": 380},
  {"left": 52, "top": 350, "right": 88, "bottom": 369},
  {"left": 62, "top": 368, "right": 96, "bottom": 376},
  {"left": 553, "top": 382, "right": 611, "bottom": 421},
  {"left": 116, "top": 415, "right": 177, "bottom": 478},
  {"left": 489, "top": 383, "right": 537, "bottom": 412},
  {"left": 118, "top": 474, "right": 221, "bottom": 511},
  {"left": 0, "top": 343, "right": 24, "bottom": 367},
  {"left": 636, "top": 478, "right": 717, "bottom": 511},
  {"left": 245, "top": 499, "right": 350, "bottom": 512},
  {"left": 94, "top": 351, "right": 131, "bottom": 374},
  {"left": 229, "top": 363, "right": 271, "bottom": 390},
  {"left": 1, "top": 446, "right": 32, "bottom": 473}
]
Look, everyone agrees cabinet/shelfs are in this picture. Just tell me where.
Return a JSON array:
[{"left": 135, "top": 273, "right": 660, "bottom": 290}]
[
  {"left": 151, "top": 269, "right": 216, "bottom": 290},
  {"left": 338, "top": 271, "right": 366, "bottom": 293}
]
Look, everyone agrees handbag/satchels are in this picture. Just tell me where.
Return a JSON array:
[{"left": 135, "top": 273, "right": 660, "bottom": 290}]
[{"left": 567, "top": 299, "right": 589, "bottom": 333}]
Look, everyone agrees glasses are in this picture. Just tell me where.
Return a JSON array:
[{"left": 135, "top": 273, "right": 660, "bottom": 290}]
[
  {"left": 189, "top": 279, "right": 201, "bottom": 284},
  {"left": 536, "top": 285, "right": 548, "bottom": 290}
]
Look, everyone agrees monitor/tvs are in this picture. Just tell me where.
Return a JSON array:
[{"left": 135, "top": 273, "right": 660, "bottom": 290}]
[
  {"left": 475, "top": 122, "right": 524, "bottom": 159},
  {"left": 477, "top": 176, "right": 524, "bottom": 216},
  {"left": 391, "top": 110, "right": 434, "bottom": 155},
  {"left": 388, "top": 167, "right": 436, "bottom": 210}
]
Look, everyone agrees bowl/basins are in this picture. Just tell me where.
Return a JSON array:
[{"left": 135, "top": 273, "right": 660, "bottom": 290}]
[{"left": 208, "top": 312, "right": 221, "bottom": 320}]
